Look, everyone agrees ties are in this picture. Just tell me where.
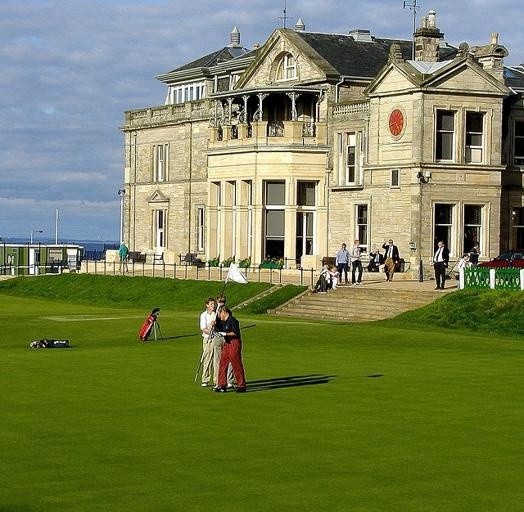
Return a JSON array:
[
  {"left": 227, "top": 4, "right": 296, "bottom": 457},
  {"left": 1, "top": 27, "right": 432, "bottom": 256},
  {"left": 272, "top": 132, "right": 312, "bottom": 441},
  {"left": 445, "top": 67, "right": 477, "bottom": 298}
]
[
  {"left": 389, "top": 248, "right": 391, "bottom": 257},
  {"left": 436, "top": 248, "right": 441, "bottom": 262},
  {"left": 377, "top": 255, "right": 378, "bottom": 262}
]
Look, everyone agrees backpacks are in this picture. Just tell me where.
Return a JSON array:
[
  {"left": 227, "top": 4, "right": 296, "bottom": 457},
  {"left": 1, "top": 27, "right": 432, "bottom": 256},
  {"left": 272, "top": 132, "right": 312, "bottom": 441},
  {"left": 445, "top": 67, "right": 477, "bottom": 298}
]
[
  {"left": 138, "top": 308, "right": 160, "bottom": 342},
  {"left": 30, "top": 339, "right": 70, "bottom": 349}
]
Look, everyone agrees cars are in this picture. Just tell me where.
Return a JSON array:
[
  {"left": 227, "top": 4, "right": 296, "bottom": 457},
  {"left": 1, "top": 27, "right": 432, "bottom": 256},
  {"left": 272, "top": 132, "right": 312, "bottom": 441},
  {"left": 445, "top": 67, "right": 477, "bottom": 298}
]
[{"left": 477, "top": 249, "right": 524, "bottom": 268}]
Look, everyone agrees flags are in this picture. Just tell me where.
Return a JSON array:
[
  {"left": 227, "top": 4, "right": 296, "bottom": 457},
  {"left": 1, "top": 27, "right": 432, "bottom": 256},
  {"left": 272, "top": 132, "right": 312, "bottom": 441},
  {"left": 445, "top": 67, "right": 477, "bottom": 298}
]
[{"left": 226, "top": 263, "right": 251, "bottom": 285}]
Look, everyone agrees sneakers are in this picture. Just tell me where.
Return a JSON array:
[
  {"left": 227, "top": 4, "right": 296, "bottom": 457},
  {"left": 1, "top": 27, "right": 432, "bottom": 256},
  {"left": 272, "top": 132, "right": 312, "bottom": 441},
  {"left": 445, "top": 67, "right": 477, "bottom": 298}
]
[
  {"left": 201, "top": 383, "right": 246, "bottom": 392},
  {"left": 352, "top": 281, "right": 362, "bottom": 285}
]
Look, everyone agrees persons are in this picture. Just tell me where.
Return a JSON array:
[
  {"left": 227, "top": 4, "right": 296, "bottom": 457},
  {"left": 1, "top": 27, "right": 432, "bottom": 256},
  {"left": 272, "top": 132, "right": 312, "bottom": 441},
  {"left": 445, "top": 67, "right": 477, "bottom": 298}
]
[
  {"left": 118, "top": 239, "right": 130, "bottom": 272},
  {"left": 312, "top": 239, "right": 400, "bottom": 294},
  {"left": 458, "top": 242, "right": 481, "bottom": 270},
  {"left": 199, "top": 296, "right": 246, "bottom": 393},
  {"left": 433, "top": 240, "right": 449, "bottom": 290}
]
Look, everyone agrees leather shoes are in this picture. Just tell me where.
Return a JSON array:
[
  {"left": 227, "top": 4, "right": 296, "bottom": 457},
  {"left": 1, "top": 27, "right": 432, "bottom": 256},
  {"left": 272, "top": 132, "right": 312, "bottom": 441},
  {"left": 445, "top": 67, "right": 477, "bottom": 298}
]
[{"left": 435, "top": 286, "right": 444, "bottom": 290}]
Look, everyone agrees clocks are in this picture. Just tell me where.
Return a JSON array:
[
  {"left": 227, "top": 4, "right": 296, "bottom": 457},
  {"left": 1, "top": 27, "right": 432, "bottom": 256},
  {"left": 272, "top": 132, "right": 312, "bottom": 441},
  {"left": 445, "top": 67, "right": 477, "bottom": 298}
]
[{"left": 386, "top": 105, "right": 407, "bottom": 140}]
